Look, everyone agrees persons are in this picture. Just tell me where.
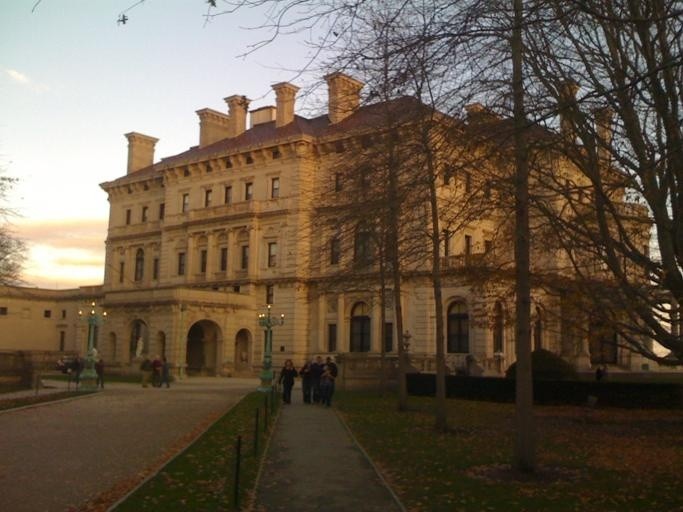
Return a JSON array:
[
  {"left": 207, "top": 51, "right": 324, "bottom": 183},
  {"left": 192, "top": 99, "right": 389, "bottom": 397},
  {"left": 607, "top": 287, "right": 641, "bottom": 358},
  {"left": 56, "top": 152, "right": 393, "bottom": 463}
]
[
  {"left": 150, "top": 355, "right": 160, "bottom": 387},
  {"left": 138, "top": 356, "right": 153, "bottom": 388},
  {"left": 93, "top": 359, "right": 106, "bottom": 389},
  {"left": 73, "top": 351, "right": 84, "bottom": 378},
  {"left": 595, "top": 365, "right": 603, "bottom": 380},
  {"left": 158, "top": 357, "right": 170, "bottom": 389},
  {"left": 299, "top": 354, "right": 337, "bottom": 408},
  {"left": 277, "top": 359, "right": 297, "bottom": 405}
]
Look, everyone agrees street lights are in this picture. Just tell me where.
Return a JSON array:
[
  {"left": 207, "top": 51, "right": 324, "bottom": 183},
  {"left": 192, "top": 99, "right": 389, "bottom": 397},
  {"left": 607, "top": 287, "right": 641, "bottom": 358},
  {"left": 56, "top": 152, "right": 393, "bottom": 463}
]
[
  {"left": 76, "top": 300, "right": 109, "bottom": 394},
  {"left": 254, "top": 302, "right": 285, "bottom": 420}
]
